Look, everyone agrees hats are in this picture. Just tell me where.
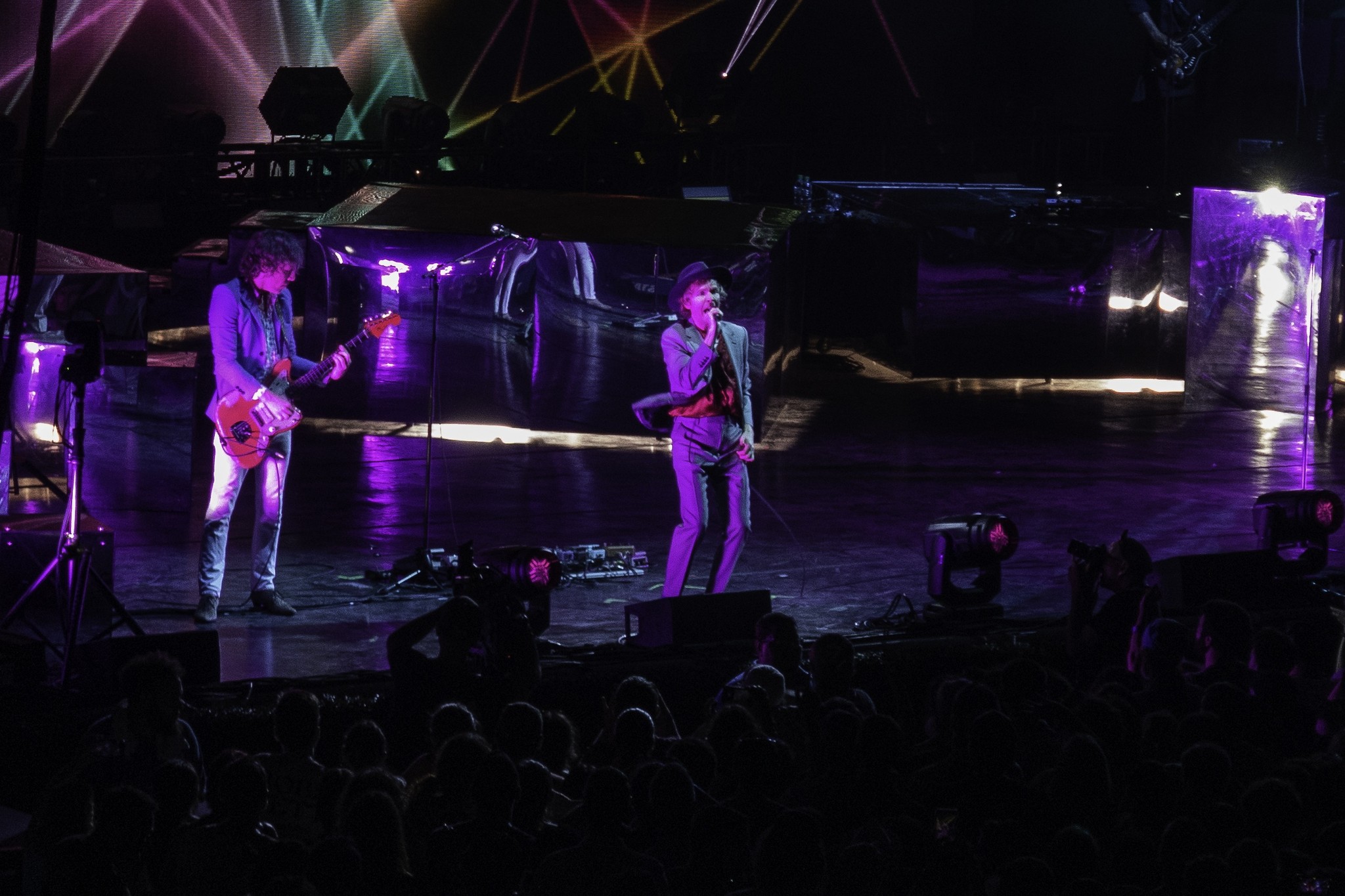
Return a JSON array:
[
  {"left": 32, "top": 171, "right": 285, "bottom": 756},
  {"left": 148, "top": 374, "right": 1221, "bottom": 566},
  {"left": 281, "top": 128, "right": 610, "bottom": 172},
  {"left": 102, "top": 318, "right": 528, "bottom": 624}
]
[{"left": 667, "top": 262, "right": 733, "bottom": 312}]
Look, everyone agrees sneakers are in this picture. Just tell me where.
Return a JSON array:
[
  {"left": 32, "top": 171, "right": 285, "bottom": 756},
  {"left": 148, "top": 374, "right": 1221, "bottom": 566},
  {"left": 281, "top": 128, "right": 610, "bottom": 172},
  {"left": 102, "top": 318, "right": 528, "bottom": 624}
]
[
  {"left": 250, "top": 589, "right": 297, "bottom": 615},
  {"left": 194, "top": 595, "right": 219, "bottom": 623}
]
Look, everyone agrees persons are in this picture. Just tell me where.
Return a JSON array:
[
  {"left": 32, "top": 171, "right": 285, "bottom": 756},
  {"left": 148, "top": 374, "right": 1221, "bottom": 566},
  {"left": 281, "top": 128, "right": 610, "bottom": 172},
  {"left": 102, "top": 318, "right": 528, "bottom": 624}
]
[
  {"left": 193, "top": 229, "right": 353, "bottom": 622},
  {"left": 23, "top": 583, "right": 1345, "bottom": 896},
  {"left": 633, "top": 261, "right": 756, "bottom": 599}
]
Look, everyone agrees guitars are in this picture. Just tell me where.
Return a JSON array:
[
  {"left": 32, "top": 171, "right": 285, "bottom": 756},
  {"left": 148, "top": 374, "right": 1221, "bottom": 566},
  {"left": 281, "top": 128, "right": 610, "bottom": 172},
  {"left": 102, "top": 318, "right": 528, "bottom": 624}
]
[{"left": 213, "top": 308, "right": 402, "bottom": 470}]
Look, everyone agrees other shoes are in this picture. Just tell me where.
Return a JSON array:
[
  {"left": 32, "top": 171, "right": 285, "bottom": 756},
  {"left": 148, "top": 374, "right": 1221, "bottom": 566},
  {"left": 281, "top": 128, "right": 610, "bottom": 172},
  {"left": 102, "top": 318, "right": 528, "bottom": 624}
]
[
  {"left": 492, "top": 313, "right": 533, "bottom": 329},
  {"left": 574, "top": 295, "right": 613, "bottom": 312}
]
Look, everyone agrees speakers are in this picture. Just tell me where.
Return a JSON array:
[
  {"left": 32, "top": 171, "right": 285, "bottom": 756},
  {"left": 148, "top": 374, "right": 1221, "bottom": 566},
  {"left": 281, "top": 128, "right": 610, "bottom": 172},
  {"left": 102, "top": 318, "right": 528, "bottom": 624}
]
[
  {"left": 68, "top": 628, "right": 221, "bottom": 694},
  {"left": 625, "top": 590, "right": 772, "bottom": 654},
  {"left": 1, "top": 504, "right": 115, "bottom": 637},
  {"left": 1151, "top": 549, "right": 1280, "bottom": 614}
]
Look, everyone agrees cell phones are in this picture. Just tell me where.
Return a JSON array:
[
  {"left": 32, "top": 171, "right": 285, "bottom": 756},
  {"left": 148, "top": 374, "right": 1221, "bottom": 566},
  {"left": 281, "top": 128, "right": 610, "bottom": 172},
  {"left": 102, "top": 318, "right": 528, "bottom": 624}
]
[{"left": 720, "top": 685, "right": 750, "bottom": 705}]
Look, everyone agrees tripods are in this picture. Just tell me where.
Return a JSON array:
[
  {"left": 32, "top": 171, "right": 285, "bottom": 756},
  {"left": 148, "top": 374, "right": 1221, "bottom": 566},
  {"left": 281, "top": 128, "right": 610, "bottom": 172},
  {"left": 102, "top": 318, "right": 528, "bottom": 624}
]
[{"left": 0, "top": 282, "right": 147, "bottom": 688}]
